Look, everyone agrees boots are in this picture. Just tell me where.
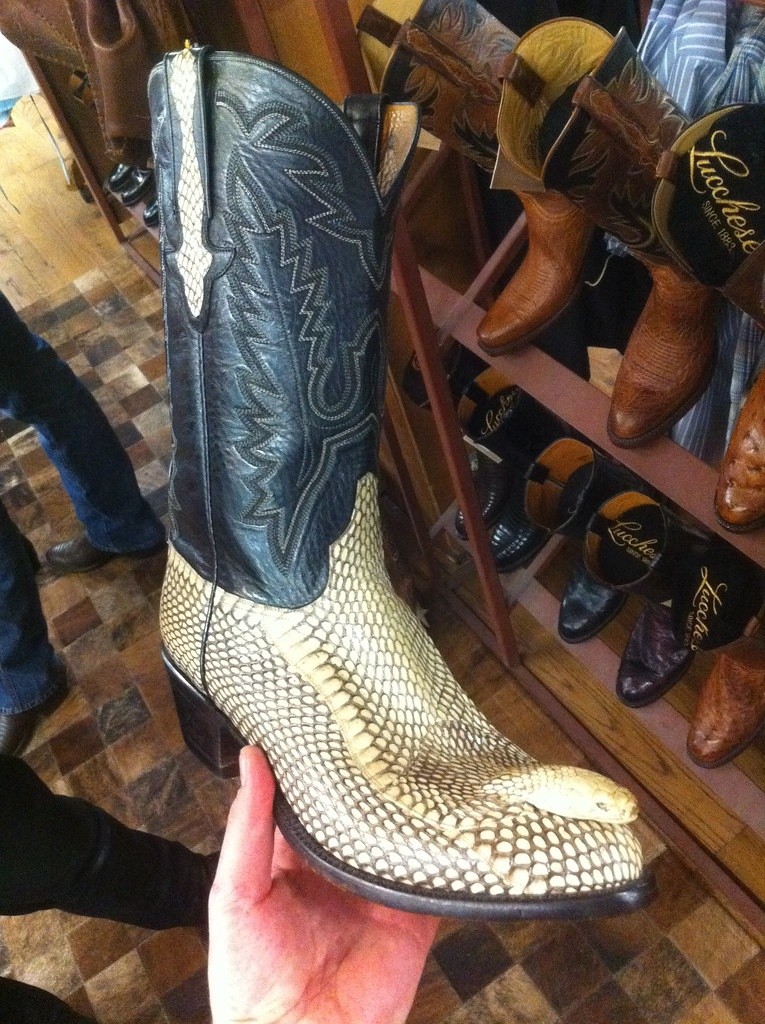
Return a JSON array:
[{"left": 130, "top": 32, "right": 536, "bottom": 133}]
[
  {"left": 650, "top": 101, "right": 765, "bottom": 532},
  {"left": 457, "top": 366, "right": 565, "bottom": 573},
  {"left": 670, "top": 541, "right": 765, "bottom": 769},
  {"left": 158, "top": 40, "right": 658, "bottom": 915},
  {"left": 401, "top": 348, "right": 505, "bottom": 541},
  {"left": 522, "top": 437, "right": 630, "bottom": 644},
  {"left": 495, "top": 16, "right": 725, "bottom": 450},
  {"left": 357, "top": 0, "right": 606, "bottom": 357},
  {"left": 584, "top": 491, "right": 707, "bottom": 708}
]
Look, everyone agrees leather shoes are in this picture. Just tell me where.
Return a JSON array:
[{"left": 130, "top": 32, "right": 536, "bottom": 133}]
[
  {"left": 0, "top": 680, "right": 69, "bottom": 758},
  {"left": 108, "top": 162, "right": 135, "bottom": 193},
  {"left": 45, "top": 534, "right": 166, "bottom": 573},
  {"left": 122, "top": 163, "right": 156, "bottom": 207},
  {"left": 143, "top": 197, "right": 158, "bottom": 226}
]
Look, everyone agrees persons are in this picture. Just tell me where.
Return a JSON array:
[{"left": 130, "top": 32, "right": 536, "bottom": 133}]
[
  {"left": 207, "top": 746, "right": 440, "bottom": 1024},
  {"left": 0, "top": 290, "right": 166, "bottom": 758},
  {"left": 0, "top": 752, "right": 219, "bottom": 1024}
]
